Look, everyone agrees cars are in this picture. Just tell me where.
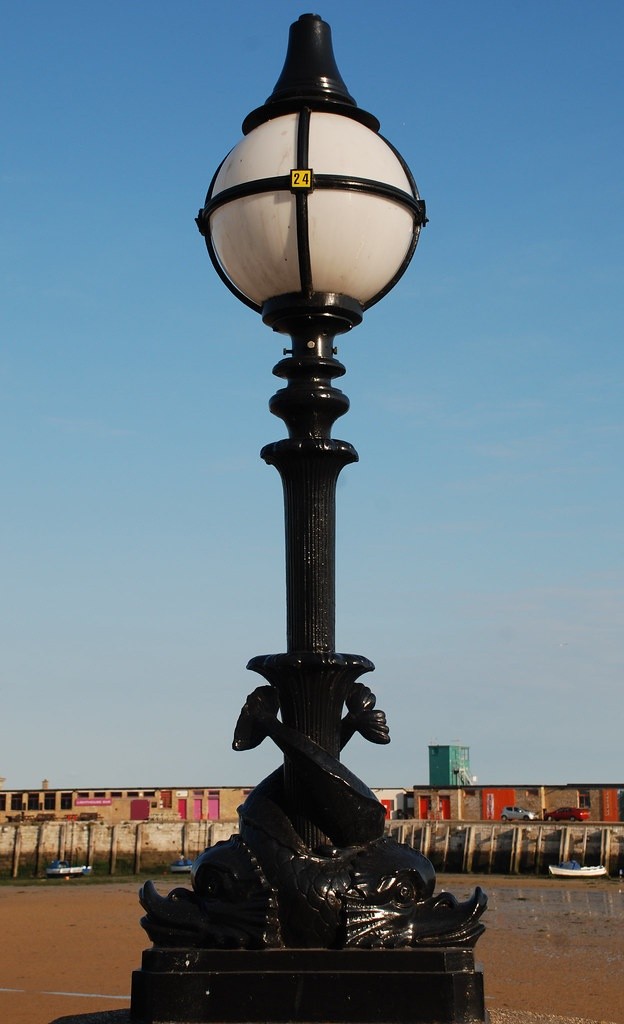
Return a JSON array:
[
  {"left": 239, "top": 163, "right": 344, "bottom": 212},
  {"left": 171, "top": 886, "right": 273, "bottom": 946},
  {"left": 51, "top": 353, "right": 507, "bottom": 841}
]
[
  {"left": 500, "top": 806, "right": 536, "bottom": 821},
  {"left": 543, "top": 806, "right": 590, "bottom": 822}
]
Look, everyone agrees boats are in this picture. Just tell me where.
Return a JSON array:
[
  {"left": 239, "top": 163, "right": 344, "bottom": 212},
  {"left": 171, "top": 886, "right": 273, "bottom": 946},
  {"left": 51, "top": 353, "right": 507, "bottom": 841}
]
[
  {"left": 44, "top": 859, "right": 93, "bottom": 879},
  {"left": 172, "top": 858, "right": 193, "bottom": 873},
  {"left": 548, "top": 860, "right": 607, "bottom": 879}
]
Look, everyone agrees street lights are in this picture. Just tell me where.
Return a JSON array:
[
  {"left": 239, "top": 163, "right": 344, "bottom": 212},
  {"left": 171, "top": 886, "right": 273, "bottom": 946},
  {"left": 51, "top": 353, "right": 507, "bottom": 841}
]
[{"left": 204, "top": 5, "right": 425, "bottom": 657}]
